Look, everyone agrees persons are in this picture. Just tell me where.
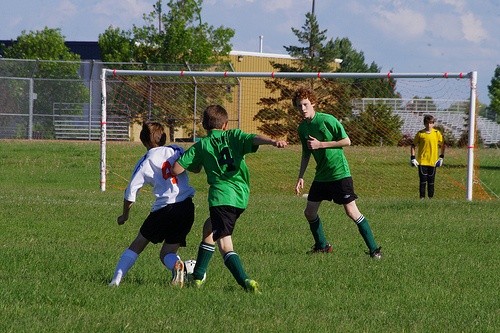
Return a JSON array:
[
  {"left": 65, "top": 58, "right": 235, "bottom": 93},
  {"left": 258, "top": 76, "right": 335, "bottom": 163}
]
[
  {"left": 172, "top": 104, "right": 289, "bottom": 295},
  {"left": 292, "top": 89, "right": 383, "bottom": 260},
  {"left": 410, "top": 115, "right": 445, "bottom": 201},
  {"left": 107, "top": 122, "right": 196, "bottom": 291}
]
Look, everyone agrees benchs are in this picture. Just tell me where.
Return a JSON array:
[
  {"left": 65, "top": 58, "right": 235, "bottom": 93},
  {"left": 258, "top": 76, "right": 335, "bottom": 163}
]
[
  {"left": 53, "top": 102, "right": 131, "bottom": 141},
  {"left": 392, "top": 111, "right": 500, "bottom": 144}
]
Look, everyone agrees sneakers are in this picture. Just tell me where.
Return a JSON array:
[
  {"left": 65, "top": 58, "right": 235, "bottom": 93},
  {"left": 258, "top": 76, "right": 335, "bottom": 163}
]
[
  {"left": 170, "top": 259, "right": 185, "bottom": 289},
  {"left": 306, "top": 242, "right": 333, "bottom": 255},
  {"left": 245, "top": 278, "right": 260, "bottom": 295},
  {"left": 191, "top": 271, "right": 207, "bottom": 288},
  {"left": 364, "top": 246, "right": 383, "bottom": 260}
]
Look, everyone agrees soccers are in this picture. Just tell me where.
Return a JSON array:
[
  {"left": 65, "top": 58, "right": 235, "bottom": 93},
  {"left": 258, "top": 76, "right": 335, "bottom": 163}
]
[{"left": 182, "top": 260, "right": 207, "bottom": 285}]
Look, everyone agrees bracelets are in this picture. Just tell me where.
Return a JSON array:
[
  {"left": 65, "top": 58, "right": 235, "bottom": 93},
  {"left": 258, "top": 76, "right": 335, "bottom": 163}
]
[
  {"left": 411, "top": 155, "right": 416, "bottom": 160},
  {"left": 439, "top": 154, "right": 444, "bottom": 158}
]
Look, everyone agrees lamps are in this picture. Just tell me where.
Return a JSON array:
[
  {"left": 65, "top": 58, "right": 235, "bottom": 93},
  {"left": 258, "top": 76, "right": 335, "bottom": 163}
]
[{"left": 238, "top": 55, "right": 243, "bottom": 62}]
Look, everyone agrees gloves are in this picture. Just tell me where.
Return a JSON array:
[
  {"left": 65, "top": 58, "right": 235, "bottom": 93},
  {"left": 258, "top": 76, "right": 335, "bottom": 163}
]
[
  {"left": 434, "top": 157, "right": 443, "bottom": 168},
  {"left": 410, "top": 158, "right": 419, "bottom": 168}
]
